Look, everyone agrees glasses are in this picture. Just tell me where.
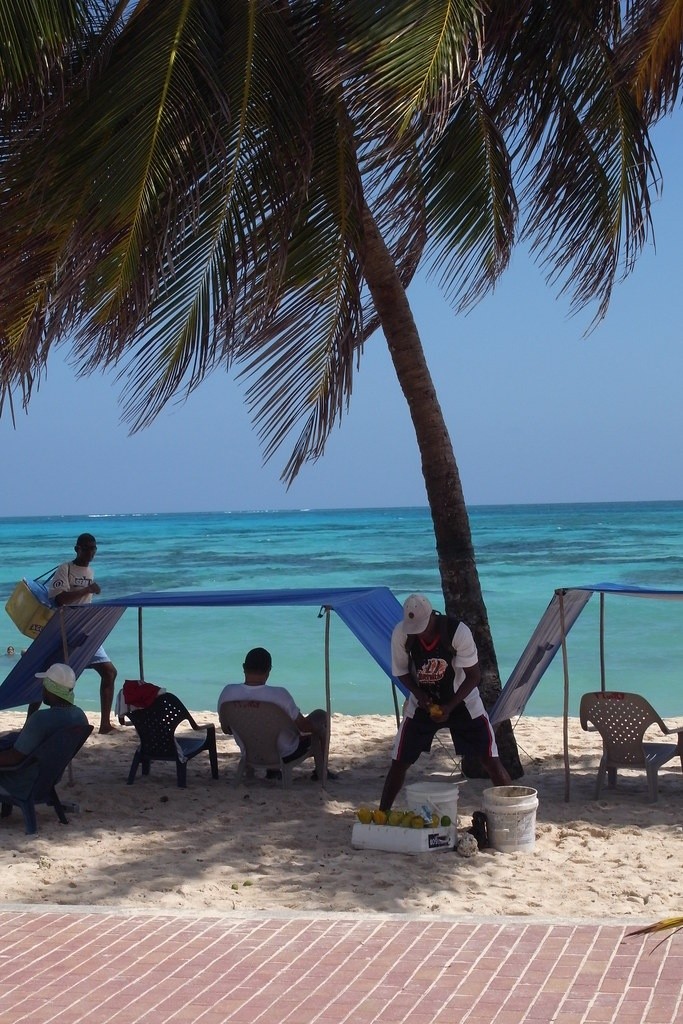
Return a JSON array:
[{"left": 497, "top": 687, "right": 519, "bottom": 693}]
[{"left": 80, "top": 546, "right": 97, "bottom": 551}]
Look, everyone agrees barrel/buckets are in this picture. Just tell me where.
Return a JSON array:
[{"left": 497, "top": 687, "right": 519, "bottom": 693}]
[
  {"left": 406, "top": 781, "right": 461, "bottom": 845},
  {"left": 482, "top": 786, "right": 539, "bottom": 848}
]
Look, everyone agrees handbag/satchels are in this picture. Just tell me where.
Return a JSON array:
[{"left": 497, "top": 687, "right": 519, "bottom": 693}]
[{"left": 454, "top": 811, "right": 487, "bottom": 850}]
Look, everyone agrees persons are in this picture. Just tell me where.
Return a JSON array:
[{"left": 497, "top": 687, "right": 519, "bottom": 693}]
[
  {"left": 377, "top": 593, "right": 513, "bottom": 813},
  {"left": 25, "top": 533, "right": 119, "bottom": 733},
  {"left": 0, "top": 663, "right": 89, "bottom": 795},
  {"left": 217, "top": 647, "right": 337, "bottom": 780},
  {"left": 6, "top": 646, "right": 15, "bottom": 655}
]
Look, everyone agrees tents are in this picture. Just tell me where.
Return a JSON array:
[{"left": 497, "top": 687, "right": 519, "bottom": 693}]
[
  {"left": 449, "top": 582, "right": 683, "bottom": 805},
  {"left": 0, "top": 585, "right": 413, "bottom": 790}
]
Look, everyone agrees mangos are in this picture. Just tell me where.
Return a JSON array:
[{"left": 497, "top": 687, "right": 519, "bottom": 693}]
[
  {"left": 358, "top": 808, "right": 439, "bottom": 829},
  {"left": 429, "top": 704, "right": 442, "bottom": 715}
]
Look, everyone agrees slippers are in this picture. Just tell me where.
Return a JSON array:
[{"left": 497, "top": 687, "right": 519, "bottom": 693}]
[
  {"left": 310, "top": 768, "right": 339, "bottom": 781},
  {"left": 264, "top": 770, "right": 282, "bottom": 781}
]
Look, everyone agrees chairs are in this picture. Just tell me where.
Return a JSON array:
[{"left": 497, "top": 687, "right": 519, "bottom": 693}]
[
  {"left": 218, "top": 699, "right": 326, "bottom": 791},
  {"left": 579, "top": 691, "right": 683, "bottom": 803},
  {"left": 0, "top": 724, "right": 95, "bottom": 834},
  {"left": 118, "top": 692, "right": 217, "bottom": 790}
]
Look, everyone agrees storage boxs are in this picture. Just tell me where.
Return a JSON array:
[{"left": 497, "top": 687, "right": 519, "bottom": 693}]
[
  {"left": 6, "top": 576, "right": 58, "bottom": 641},
  {"left": 351, "top": 823, "right": 456, "bottom": 855}
]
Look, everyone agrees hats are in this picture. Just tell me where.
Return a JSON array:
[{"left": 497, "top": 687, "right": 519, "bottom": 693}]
[
  {"left": 7, "top": 646, "right": 14, "bottom": 651},
  {"left": 403, "top": 594, "right": 433, "bottom": 634},
  {"left": 35, "top": 662, "right": 76, "bottom": 689}
]
